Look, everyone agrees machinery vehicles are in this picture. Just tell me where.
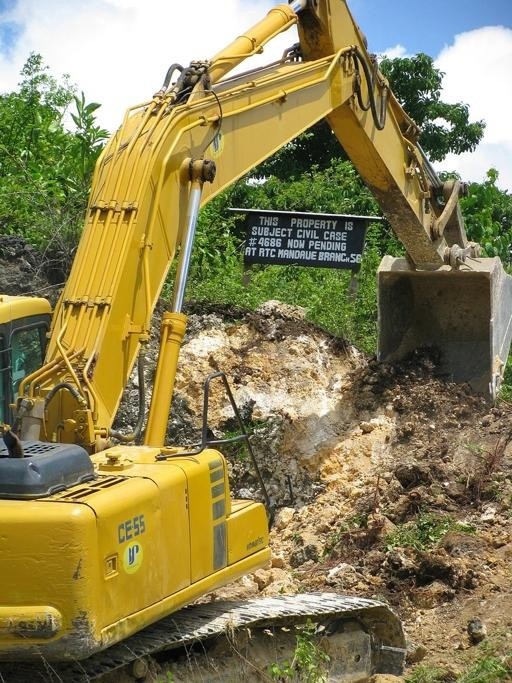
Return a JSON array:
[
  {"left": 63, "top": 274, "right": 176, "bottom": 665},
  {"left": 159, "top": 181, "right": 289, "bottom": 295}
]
[{"left": 3, "top": 3, "right": 512, "bottom": 682}]
